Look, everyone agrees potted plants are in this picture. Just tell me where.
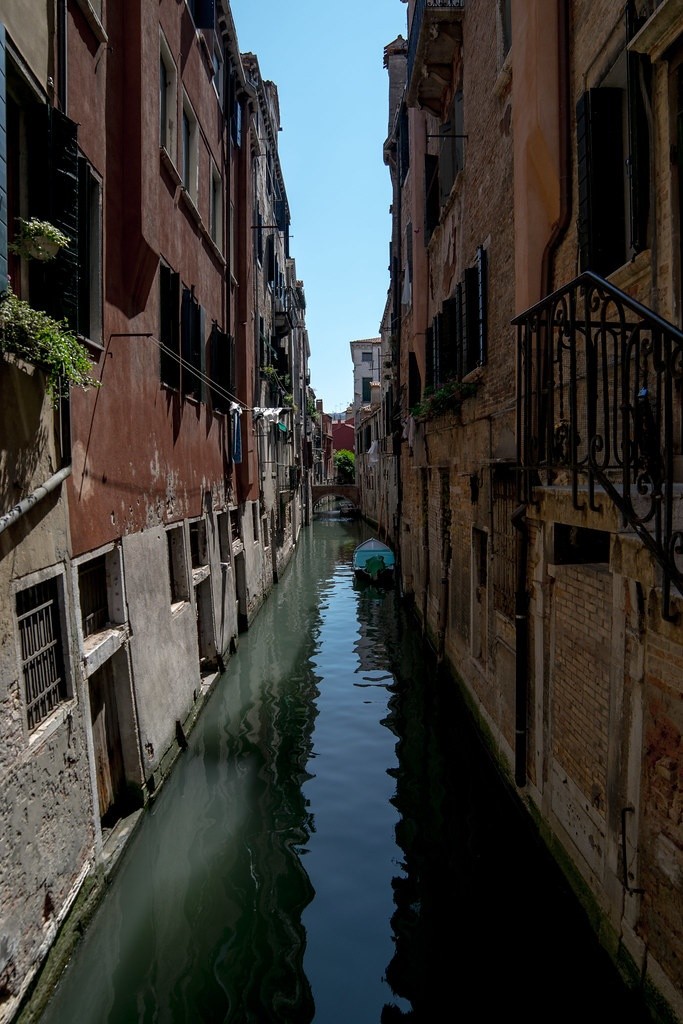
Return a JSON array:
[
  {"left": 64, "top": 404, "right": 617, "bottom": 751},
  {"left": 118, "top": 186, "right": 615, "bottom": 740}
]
[
  {"left": 0, "top": 293, "right": 104, "bottom": 415},
  {"left": 8, "top": 215, "right": 72, "bottom": 263}
]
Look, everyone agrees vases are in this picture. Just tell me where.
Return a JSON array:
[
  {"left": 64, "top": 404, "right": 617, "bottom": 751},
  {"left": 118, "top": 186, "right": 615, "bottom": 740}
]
[{"left": 454, "top": 390, "right": 466, "bottom": 400}]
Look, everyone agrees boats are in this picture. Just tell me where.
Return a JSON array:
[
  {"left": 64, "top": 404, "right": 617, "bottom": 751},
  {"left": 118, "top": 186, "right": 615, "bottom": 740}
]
[{"left": 353, "top": 537, "right": 397, "bottom": 583}]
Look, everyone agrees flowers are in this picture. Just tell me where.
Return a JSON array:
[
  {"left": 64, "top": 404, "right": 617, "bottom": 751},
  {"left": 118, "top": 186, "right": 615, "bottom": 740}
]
[{"left": 410, "top": 373, "right": 477, "bottom": 421}]
